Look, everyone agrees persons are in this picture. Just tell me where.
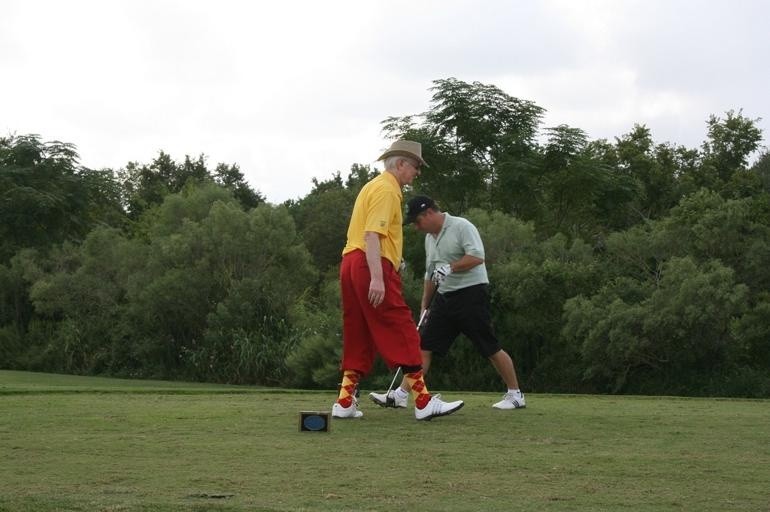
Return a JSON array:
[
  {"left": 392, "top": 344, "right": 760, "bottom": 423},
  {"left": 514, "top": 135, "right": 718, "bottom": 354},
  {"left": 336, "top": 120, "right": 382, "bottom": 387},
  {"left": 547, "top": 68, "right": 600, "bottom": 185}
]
[
  {"left": 331, "top": 139, "right": 465, "bottom": 422},
  {"left": 369, "top": 195, "right": 527, "bottom": 410}
]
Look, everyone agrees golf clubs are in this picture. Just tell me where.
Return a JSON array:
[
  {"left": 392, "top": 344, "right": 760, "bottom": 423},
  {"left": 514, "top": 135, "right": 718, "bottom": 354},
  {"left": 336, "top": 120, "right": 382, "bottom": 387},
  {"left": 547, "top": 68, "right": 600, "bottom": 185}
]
[{"left": 385, "top": 281, "right": 440, "bottom": 408}]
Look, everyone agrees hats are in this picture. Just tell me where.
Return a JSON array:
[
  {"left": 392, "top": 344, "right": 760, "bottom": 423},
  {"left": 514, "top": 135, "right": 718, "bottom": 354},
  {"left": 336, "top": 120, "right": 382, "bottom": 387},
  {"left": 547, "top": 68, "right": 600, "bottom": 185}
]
[
  {"left": 402, "top": 196, "right": 435, "bottom": 227},
  {"left": 377, "top": 140, "right": 430, "bottom": 169}
]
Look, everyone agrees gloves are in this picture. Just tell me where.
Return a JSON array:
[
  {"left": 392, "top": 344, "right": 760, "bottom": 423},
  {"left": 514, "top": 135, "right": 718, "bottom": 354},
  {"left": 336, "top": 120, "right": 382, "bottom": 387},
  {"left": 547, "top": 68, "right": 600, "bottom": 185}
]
[{"left": 431, "top": 263, "right": 454, "bottom": 286}]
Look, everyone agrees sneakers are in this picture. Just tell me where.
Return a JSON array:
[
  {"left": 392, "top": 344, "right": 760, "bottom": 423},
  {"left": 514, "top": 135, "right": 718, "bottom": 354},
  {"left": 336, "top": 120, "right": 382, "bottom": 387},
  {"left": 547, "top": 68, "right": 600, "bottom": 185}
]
[
  {"left": 332, "top": 401, "right": 363, "bottom": 418},
  {"left": 492, "top": 392, "right": 526, "bottom": 410},
  {"left": 414, "top": 393, "right": 464, "bottom": 421},
  {"left": 369, "top": 389, "right": 408, "bottom": 408}
]
